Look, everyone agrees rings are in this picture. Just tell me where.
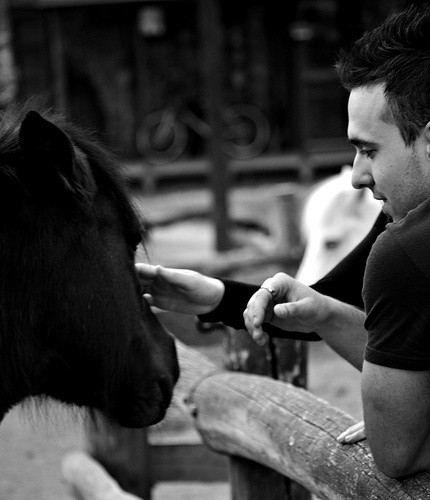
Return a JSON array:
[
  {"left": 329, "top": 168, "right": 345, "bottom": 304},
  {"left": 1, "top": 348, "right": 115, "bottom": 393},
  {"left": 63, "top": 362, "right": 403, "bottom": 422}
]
[{"left": 259, "top": 283, "right": 275, "bottom": 297}]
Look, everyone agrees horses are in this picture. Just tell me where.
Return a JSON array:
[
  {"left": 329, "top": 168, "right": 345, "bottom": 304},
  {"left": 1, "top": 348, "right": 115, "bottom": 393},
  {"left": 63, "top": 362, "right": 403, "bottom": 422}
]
[
  {"left": 0, "top": 93, "right": 181, "bottom": 428},
  {"left": 293, "top": 164, "right": 385, "bottom": 288}
]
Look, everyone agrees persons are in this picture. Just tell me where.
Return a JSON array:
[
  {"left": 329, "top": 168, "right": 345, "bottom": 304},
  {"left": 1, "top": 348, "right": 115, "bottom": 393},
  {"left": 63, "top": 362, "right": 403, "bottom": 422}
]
[
  {"left": 132, "top": 207, "right": 391, "bottom": 445},
  {"left": 243, "top": 2, "right": 430, "bottom": 477}
]
